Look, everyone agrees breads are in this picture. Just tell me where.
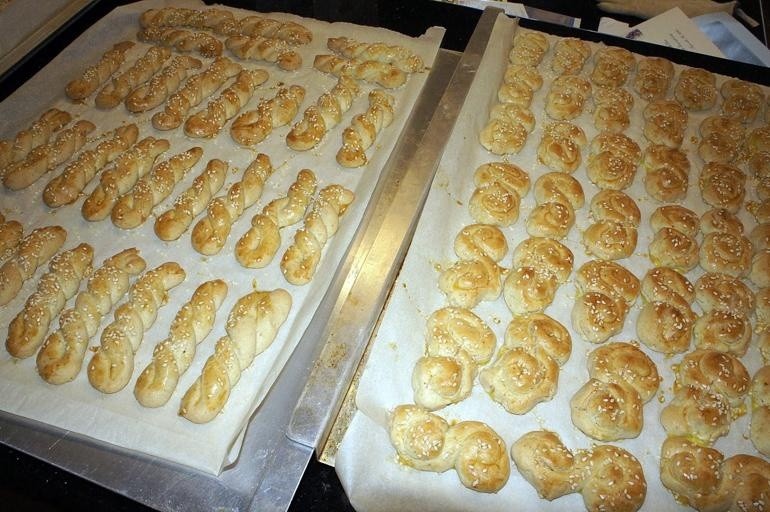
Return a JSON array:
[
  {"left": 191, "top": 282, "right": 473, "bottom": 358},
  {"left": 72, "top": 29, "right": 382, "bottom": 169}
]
[
  {"left": 391, "top": 31, "right": 767, "bottom": 510},
  {"left": 0, "top": 7, "right": 422, "bottom": 430}
]
